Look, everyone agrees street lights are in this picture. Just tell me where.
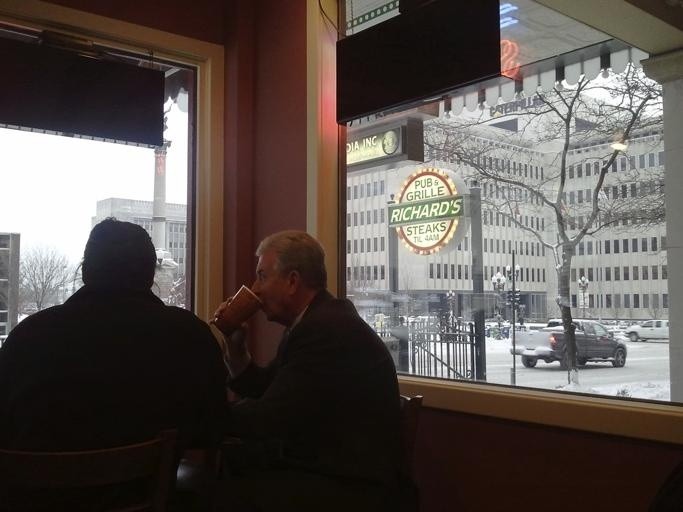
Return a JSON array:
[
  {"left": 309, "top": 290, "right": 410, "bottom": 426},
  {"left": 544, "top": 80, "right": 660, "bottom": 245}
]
[
  {"left": 506, "top": 249, "right": 522, "bottom": 386},
  {"left": 150, "top": 88, "right": 187, "bottom": 309},
  {"left": 446, "top": 289, "right": 455, "bottom": 327},
  {"left": 490, "top": 272, "right": 506, "bottom": 336},
  {"left": 577, "top": 276, "right": 589, "bottom": 318}
]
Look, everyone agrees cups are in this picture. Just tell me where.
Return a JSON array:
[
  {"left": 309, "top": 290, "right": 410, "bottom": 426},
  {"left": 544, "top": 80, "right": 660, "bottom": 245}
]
[{"left": 211, "top": 285, "right": 263, "bottom": 337}]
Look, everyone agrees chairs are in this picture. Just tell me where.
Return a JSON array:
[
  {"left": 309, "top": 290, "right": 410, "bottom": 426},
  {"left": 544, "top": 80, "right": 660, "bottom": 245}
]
[
  {"left": 0, "top": 428, "right": 177, "bottom": 512},
  {"left": 399, "top": 394, "right": 423, "bottom": 479}
]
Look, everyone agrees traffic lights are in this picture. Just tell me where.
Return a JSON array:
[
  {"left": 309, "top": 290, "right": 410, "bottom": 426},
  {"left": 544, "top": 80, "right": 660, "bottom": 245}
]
[
  {"left": 506, "top": 290, "right": 511, "bottom": 303},
  {"left": 515, "top": 289, "right": 520, "bottom": 301}
]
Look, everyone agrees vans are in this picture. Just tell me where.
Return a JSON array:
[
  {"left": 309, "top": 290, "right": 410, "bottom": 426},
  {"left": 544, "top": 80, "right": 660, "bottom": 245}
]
[{"left": 623, "top": 319, "right": 669, "bottom": 343}]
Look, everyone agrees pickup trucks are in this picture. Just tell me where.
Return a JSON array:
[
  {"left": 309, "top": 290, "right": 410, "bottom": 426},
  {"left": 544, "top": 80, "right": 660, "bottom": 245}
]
[{"left": 508, "top": 318, "right": 629, "bottom": 373}]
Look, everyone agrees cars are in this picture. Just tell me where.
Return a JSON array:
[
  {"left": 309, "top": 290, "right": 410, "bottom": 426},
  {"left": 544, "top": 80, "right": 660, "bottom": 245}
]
[
  {"left": 366, "top": 312, "right": 439, "bottom": 324},
  {"left": 485, "top": 318, "right": 509, "bottom": 330}
]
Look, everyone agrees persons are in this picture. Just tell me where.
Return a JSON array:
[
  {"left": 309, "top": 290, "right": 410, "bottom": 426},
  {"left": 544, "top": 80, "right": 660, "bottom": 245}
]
[
  {"left": 399, "top": 316, "right": 406, "bottom": 328},
  {"left": 0, "top": 217, "right": 228, "bottom": 511},
  {"left": 206, "top": 229, "right": 422, "bottom": 511},
  {"left": 517, "top": 314, "right": 524, "bottom": 329}
]
